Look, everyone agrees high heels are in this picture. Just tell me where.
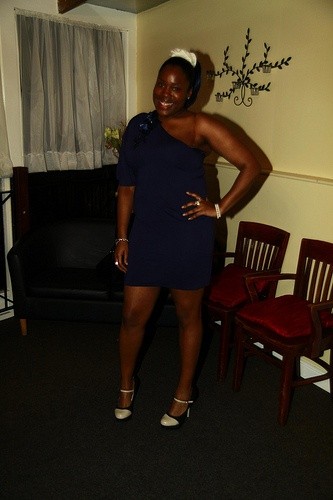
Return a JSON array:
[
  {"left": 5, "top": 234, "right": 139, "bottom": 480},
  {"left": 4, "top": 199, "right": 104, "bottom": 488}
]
[
  {"left": 114, "top": 389, "right": 135, "bottom": 421},
  {"left": 160, "top": 397, "right": 194, "bottom": 428}
]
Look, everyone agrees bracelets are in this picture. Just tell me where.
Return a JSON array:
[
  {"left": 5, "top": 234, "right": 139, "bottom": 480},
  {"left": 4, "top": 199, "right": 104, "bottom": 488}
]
[
  {"left": 214, "top": 202, "right": 222, "bottom": 219},
  {"left": 115, "top": 239, "right": 128, "bottom": 245}
]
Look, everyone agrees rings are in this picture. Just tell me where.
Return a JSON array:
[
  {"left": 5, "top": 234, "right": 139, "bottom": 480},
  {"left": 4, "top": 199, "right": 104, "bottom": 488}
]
[
  {"left": 195, "top": 200, "right": 200, "bottom": 207},
  {"left": 114, "top": 261, "right": 117, "bottom": 265}
]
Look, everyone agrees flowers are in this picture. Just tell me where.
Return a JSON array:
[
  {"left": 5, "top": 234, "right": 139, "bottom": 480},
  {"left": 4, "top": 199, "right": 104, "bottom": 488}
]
[{"left": 104, "top": 127, "right": 122, "bottom": 158}]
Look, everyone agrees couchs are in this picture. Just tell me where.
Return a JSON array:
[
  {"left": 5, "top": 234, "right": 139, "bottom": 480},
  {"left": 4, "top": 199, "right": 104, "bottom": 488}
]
[{"left": 7, "top": 216, "right": 178, "bottom": 337}]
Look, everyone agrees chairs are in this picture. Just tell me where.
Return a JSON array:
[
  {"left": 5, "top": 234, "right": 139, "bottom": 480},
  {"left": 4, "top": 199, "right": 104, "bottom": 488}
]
[
  {"left": 233, "top": 239, "right": 333, "bottom": 424},
  {"left": 200, "top": 222, "right": 290, "bottom": 382}
]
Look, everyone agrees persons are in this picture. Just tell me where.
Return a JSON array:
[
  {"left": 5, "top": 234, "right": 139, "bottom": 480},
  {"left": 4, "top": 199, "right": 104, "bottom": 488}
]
[{"left": 115, "top": 47, "right": 261, "bottom": 430}]
